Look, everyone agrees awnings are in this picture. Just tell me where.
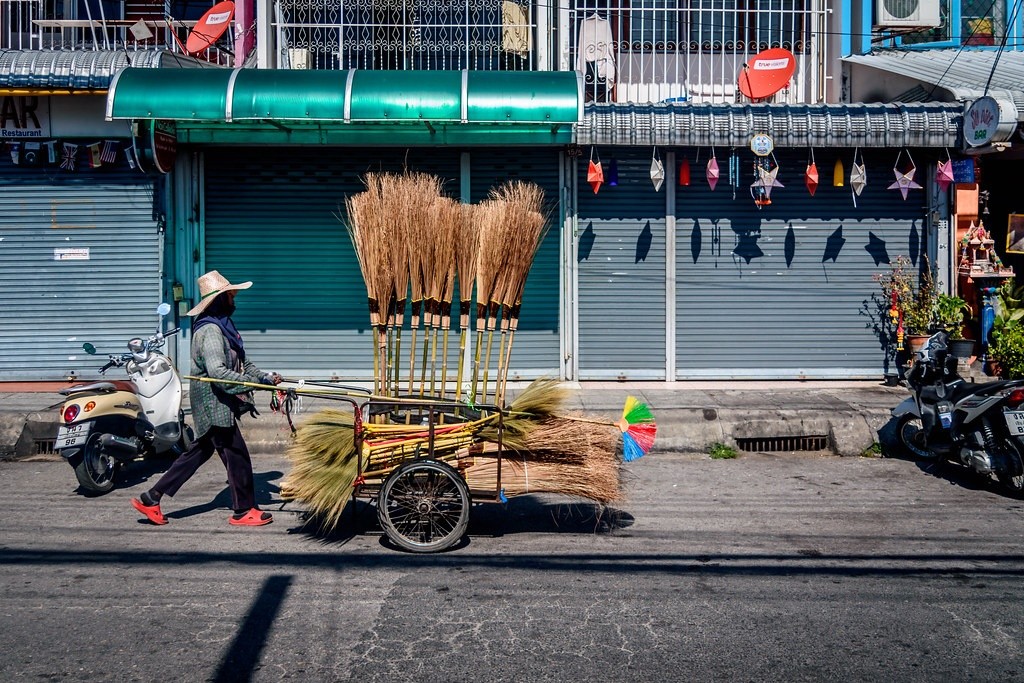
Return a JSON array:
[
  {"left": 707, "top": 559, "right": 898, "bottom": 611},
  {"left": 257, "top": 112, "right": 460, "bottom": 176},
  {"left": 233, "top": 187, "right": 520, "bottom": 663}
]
[{"left": 105, "top": 66, "right": 584, "bottom": 128}]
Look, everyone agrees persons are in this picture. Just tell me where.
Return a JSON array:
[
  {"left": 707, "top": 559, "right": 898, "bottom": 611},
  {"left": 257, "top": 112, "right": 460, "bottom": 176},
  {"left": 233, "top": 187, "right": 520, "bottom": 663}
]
[{"left": 132, "top": 270, "right": 283, "bottom": 526}]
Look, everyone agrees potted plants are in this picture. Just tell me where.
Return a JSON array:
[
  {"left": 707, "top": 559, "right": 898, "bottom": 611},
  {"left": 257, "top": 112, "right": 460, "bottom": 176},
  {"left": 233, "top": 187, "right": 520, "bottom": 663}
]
[{"left": 938, "top": 293, "right": 977, "bottom": 358}]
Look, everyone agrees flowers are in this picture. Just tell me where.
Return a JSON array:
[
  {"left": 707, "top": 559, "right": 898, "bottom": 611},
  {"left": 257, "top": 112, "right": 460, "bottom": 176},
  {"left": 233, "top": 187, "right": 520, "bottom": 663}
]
[{"left": 871, "top": 253, "right": 943, "bottom": 336}]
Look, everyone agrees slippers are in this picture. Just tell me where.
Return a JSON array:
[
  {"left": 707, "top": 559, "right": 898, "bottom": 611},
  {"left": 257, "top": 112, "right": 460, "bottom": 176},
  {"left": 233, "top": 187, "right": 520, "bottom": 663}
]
[
  {"left": 132, "top": 498, "right": 169, "bottom": 525},
  {"left": 230, "top": 507, "right": 273, "bottom": 526}
]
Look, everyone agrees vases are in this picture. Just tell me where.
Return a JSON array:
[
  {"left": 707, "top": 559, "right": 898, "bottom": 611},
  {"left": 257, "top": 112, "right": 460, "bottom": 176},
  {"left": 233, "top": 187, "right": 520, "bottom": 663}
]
[{"left": 908, "top": 335, "right": 931, "bottom": 356}]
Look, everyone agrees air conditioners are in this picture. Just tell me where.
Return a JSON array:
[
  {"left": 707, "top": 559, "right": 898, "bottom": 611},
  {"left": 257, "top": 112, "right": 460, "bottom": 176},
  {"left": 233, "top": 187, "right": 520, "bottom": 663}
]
[{"left": 872, "top": 0, "right": 941, "bottom": 32}]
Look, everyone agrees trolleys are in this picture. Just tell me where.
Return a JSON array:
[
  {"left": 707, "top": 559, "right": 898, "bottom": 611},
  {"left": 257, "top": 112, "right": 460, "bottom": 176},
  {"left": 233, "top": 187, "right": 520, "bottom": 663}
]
[{"left": 260, "top": 377, "right": 504, "bottom": 553}]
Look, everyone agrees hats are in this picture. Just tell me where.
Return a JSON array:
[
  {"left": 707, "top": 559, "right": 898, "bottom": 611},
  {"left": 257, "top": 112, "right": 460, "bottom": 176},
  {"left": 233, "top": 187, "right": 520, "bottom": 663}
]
[{"left": 186, "top": 270, "right": 254, "bottom": 316}]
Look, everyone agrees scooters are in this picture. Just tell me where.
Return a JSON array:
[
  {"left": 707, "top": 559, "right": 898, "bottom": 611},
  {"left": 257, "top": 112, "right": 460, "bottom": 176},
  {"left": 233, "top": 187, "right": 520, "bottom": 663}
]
[{"left": 53, "top": 304, "right": 193, "bottom": 496}]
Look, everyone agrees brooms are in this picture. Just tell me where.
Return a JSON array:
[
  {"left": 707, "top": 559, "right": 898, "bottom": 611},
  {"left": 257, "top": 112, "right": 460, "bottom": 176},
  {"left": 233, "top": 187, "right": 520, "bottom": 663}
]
[{"left": 279, "top": 149, "right": 657, "bottom": 534}]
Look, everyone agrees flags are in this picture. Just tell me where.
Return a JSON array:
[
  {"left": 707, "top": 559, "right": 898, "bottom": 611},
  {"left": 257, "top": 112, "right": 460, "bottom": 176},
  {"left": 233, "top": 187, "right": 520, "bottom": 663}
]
[
  {"left": 59, "top": 142, "right": 78, "bottom": 171},
  {"left": 5, "top": 142, "right": 20, "bottom": 164},
  {"left": 100, "top": 140, "right": 120, "bottom": 164},
  {"left": 125, "top": 146, "right": 142, "bottom": 169},
  {"left": 86, "top": 142, "right": 104, "bottom": 168},
  {"left": 43, "top": 141, "right": 61, "bottom": 166},
  {"left": 24, "top": 142, "right": 40, "bottom": 165}
]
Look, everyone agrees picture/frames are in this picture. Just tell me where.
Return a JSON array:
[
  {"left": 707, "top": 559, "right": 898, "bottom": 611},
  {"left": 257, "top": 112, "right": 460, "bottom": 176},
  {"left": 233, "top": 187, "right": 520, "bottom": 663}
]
[{"left": 1006, "top": 214, "right": 1024, "bottom": 254}]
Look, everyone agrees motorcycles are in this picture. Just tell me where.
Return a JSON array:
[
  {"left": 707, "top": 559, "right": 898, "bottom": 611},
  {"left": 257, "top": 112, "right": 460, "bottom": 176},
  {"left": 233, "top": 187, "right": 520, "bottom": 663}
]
[{"left": 889, "top": 320, "right": 1024, "bottom": 497}]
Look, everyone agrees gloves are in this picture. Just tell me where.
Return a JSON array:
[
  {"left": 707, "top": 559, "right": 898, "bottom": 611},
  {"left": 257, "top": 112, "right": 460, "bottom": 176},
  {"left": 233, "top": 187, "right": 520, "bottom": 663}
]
[{"left": 263, "top": 372, "right": 284, "bottom": 385}]
